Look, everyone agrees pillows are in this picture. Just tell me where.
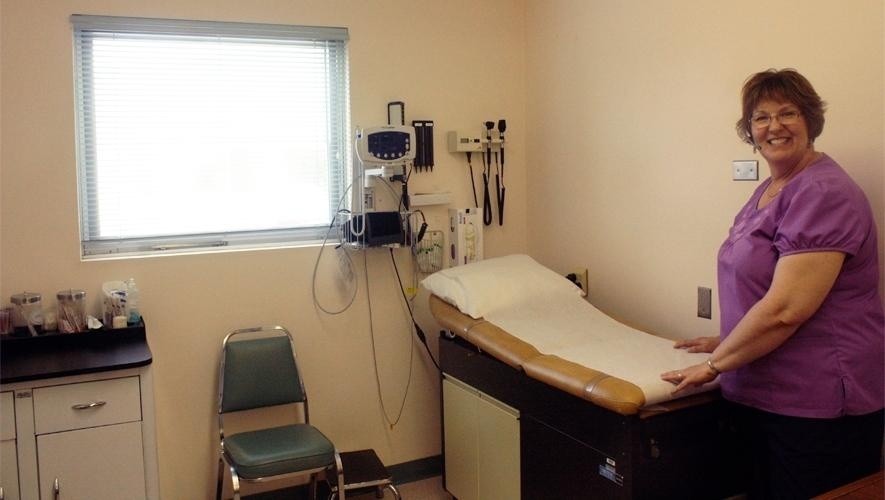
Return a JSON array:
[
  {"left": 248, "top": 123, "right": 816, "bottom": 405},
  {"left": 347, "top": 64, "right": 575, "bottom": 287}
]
[{"left": 419, "top": 253, "right": 588, "bottom": 320}]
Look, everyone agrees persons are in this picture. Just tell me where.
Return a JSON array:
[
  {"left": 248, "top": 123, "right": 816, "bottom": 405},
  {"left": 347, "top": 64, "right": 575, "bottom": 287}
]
[{"left": 660, "top": 67, "right": 884, "bottom": 499}]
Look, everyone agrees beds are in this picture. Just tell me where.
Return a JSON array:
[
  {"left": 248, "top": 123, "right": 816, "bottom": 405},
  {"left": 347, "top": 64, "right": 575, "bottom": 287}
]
[{"left": 417, "top": 254, "right": 742, "bottom": 499}]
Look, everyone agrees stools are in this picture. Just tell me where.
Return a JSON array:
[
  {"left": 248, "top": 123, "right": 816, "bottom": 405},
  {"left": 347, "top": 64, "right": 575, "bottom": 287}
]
[{"left": 328, "top": 449, "right": 402, "bottom": 500}]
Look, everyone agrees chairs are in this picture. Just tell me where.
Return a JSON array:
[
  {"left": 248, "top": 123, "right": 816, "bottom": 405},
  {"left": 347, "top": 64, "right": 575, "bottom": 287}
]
[{"left": 216, "top": 326, "right": 344, "bottom": 499}]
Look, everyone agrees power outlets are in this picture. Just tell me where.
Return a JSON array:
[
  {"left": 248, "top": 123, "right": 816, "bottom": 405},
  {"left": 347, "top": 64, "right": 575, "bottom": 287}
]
[{"left": 566, "top": 269, "right": 588, "bottom": 297}]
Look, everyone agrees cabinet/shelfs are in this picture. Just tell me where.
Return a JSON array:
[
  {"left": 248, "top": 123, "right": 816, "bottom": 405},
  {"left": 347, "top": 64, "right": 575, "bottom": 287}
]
[
  {"left": 31, "top": 358, "right": 161, "bottom": 499},
  {"left": 0, "top": 379, "right": 21, "bottom": 499}
]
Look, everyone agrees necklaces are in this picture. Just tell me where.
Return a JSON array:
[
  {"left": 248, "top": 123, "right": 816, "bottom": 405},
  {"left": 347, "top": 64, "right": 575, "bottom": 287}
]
[{"left": 766, "top": 179, "right": 782, "bottom": 200}]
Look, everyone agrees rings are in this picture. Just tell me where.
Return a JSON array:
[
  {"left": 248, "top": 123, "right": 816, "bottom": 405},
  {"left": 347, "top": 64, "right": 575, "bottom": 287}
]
[{"left": 678, "top": 372, "right": 682, "bottom": 379}]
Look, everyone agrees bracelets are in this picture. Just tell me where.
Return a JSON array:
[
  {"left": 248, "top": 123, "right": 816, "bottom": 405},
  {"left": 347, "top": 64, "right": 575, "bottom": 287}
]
[{"left": 705, "top": 356, "right": 721, "bottom": 379}]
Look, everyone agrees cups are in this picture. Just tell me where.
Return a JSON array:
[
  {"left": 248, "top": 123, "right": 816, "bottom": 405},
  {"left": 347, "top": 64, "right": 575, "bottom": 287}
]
[
  {"left": 0, "top": 310, "right": 9, "bottom": 334},
  {"left": 5, "top": 304, "right": 15, "bottom": 333}
]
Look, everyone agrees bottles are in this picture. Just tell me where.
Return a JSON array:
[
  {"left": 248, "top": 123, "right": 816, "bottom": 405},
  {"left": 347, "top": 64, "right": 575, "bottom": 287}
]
[
  {"left": 126, "top": 277, "right": 141, "bottom": 324},
  {"left": 56, "top": 288, "right": 88, "bottom": 334},
  {"left": 10, "top": 291, "right": 43, "bottom": 338},
  {"left": 102, "top": 280, "right": 128, "bottom": 329}
]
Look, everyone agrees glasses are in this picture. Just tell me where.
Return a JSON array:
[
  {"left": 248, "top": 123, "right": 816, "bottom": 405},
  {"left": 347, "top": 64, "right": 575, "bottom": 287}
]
[{"left": 748, "top": 111, "right": 799, "bottom": 128}]
